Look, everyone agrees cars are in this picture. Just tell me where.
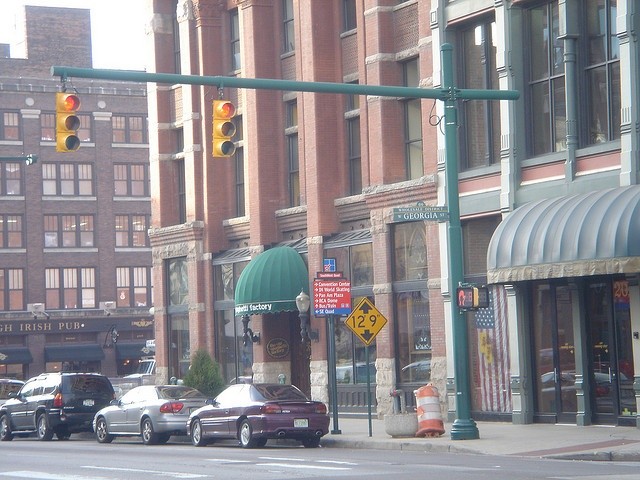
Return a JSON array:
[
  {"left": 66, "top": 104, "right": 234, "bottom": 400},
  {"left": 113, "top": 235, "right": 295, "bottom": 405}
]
[
  {"left": 92, "top": 384, "right": 212, "bottom": 445},
  {"left": 186, "top": 382, "right": 331, "bottom": 448}
]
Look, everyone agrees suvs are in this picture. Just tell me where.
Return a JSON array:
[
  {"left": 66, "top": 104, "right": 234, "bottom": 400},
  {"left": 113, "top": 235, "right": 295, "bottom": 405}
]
[{"left": 0, "top": 372, "right": 117, "bottom": 440}]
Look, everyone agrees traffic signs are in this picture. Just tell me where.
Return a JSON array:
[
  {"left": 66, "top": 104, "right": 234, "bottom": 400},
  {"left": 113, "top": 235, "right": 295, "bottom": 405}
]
[
  {"left": 313, "top": 279, "right": 352, "bottom": 316},
  {"left": 393, "top": 207, "right": 449, "bottom": 222}
]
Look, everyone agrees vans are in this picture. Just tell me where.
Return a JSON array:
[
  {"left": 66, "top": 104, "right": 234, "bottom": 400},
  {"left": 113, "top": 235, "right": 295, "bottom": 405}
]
[{"left": 0, "top": 379, "right": 24, "bottom": 410}]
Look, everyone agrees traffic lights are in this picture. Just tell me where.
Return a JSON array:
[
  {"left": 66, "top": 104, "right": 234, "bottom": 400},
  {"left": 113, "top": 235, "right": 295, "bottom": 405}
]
[
  {"left": 55, "top": 92, "right": 81, "bottom": 153},
  {"left": 211, "top": 100, "right": 236, "bottom": 158},
  {"left": 25, "top": 153, "right": 38, "bottom": 167},
  {"left": 456, "top": 285, "right": 475, "bottom": 310}
]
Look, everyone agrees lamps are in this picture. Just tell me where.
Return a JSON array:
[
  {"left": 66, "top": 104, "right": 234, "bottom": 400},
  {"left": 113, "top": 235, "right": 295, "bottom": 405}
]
[
  {"left": 103, "top": 324, "right": 120, "bottom": 349},
  {"left": 296, "top": 287, "right": 320, "bottom": 344}
]
